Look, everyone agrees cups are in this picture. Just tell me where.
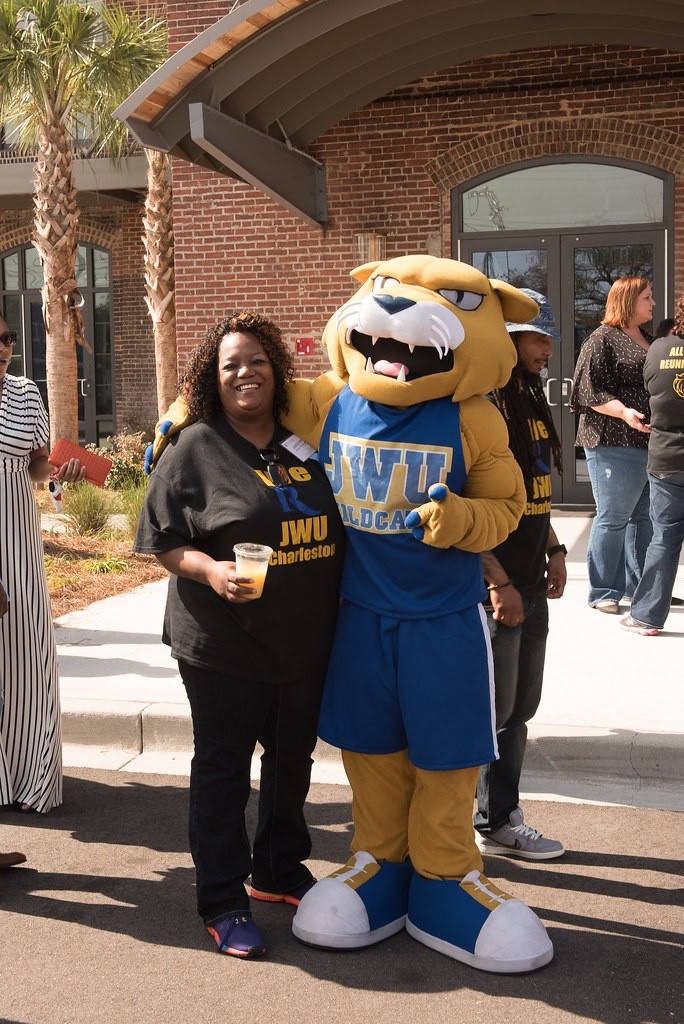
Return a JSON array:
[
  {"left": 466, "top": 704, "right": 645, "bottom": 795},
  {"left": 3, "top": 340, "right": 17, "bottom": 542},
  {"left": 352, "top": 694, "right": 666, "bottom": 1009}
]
[{"left": 232, "top": 543, "right": 273, "bottom": 599}]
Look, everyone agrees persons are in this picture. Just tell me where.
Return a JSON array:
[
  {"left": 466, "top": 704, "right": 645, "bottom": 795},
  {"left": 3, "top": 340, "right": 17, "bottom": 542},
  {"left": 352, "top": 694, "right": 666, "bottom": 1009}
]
[
  {"left": 655, "top": 318, "right": 675, "bottom": 338},
  {"left": 616, "top": 291, "right": 683, "bottom": 632},
  {"left": 470, "top": 288, "right": 568, "bottom": 859},
  {"left": 571, "top": 277, "right": 654, "bottom": 614},
  {"left": 0, "top": 314, "right": 86, "bottom": 815},
  {"left": 133, "top": 313, "right": 348, "bottom": 960}
]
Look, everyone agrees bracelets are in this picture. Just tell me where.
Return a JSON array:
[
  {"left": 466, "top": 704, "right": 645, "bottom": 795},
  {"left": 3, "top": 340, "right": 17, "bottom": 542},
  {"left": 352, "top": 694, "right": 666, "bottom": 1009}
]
[
  {"left": 50, "top": 470, "right": 62, "bottom": 481},
  {"left": 487, "top": 579, "right": 512, "bottom": 591},
  {"left": 548, "top": 544, "right": 567, "bottom": 559}
]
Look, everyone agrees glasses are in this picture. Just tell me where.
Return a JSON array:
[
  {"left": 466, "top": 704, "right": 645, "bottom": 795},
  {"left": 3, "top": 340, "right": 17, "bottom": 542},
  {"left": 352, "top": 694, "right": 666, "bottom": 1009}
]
[
  {"left": 261, "top": 447, "right": 288, "bottom": 488},
  {"left": 0, "top": 331, "right": 16, "bottom": 347}
]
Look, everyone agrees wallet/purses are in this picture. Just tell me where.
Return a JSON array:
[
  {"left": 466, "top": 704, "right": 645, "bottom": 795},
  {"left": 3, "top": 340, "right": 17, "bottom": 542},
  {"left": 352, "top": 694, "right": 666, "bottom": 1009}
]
[{"left": 47, "top": 439, "right": 112, "bottom": 485}]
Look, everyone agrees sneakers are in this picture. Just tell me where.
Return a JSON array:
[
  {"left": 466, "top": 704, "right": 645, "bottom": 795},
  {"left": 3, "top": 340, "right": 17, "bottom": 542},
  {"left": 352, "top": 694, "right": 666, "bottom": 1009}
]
[
  {"left": 249, "top": 861, "right": 320, "bottom": 905},
  {"left": 619, "top": 615, "right": 659, "bottom": 636},
  {"left": 208, "top": 914, "right": 266, "bottom": 959},
  {"left": 472, "top": 806, "right": 564, "bottom": 860}
]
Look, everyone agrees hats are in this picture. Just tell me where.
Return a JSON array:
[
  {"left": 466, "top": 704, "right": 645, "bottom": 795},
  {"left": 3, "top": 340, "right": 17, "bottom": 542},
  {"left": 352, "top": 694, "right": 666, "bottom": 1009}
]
[{"left": 500, "top": 287, "right": 556, "bottom": 338}]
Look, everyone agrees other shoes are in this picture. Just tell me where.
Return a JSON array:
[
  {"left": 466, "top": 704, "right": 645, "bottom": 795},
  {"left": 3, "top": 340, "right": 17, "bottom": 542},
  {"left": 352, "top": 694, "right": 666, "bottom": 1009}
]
[{"left": 597, "top": 601, "right": 620, "bottom": 613}]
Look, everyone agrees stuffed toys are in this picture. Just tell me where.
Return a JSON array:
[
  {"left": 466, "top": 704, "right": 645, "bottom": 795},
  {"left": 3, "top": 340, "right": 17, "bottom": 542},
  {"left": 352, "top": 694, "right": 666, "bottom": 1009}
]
[{"left": 140, "top": 255, "right": 555, "bottom": 975}]
